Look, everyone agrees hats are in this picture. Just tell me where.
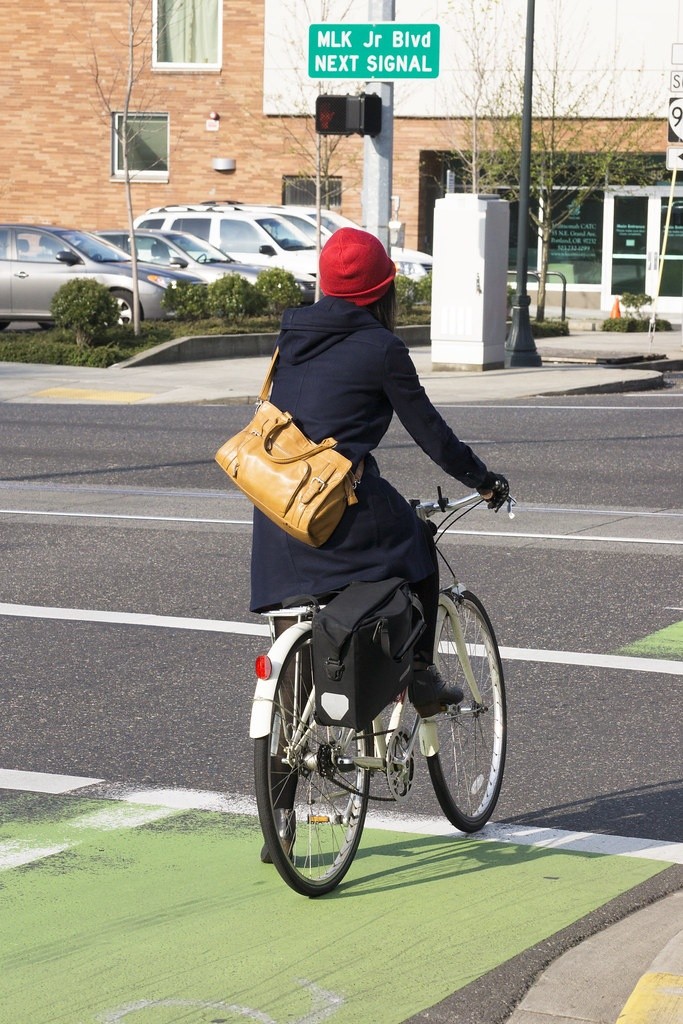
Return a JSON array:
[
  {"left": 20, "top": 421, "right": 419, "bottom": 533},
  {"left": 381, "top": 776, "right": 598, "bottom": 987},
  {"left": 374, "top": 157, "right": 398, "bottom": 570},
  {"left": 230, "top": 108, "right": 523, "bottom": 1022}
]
[{"left": 319, "top": 227, "right": 396, "bottom": 306}]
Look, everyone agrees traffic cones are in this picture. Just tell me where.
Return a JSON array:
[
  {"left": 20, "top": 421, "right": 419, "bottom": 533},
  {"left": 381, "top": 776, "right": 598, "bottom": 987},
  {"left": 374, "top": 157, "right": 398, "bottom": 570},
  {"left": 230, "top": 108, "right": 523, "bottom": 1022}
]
[{"left": 610, "top": 298, "right": 621, "bottom": 318}]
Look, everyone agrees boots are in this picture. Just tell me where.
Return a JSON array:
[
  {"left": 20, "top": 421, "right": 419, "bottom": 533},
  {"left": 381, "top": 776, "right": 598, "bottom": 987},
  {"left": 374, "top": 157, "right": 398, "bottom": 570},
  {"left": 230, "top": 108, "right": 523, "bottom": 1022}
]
[
  {"left": 408, "top": 663, "right": 464, "bottom": 718},
  {"left": 261, "top": 808, "right": 296, "bottom": 863}
]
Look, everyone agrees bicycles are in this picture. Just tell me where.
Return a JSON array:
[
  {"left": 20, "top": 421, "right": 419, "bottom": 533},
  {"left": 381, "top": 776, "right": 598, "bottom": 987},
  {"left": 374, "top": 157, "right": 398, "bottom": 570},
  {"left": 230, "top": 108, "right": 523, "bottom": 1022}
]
[{"left": 246, "top": 481, "right": 518, "bottom": 899}]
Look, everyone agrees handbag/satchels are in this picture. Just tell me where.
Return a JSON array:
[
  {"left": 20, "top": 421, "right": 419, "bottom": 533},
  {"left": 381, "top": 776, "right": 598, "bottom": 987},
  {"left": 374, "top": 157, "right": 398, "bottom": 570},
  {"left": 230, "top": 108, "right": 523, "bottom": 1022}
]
[
  {"left": 281, "top": 577, "right": 427, "bottom": 732},
  {"left": 215, "top": 400, "right": 358, "bottom": 547}
]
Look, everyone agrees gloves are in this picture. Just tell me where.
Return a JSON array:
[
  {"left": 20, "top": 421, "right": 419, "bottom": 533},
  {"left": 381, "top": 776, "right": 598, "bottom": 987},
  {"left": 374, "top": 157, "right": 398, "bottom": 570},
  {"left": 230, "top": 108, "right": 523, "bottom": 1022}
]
[{"left": 476, "top": 472, "right": 509, "bottom": 513}]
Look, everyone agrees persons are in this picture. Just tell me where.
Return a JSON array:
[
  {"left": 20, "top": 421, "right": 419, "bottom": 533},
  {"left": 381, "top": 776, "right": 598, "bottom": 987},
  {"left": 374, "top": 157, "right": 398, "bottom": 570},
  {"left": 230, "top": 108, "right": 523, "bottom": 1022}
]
[{"left": 249, "top": 228, "right": 511, "bottom": 864}]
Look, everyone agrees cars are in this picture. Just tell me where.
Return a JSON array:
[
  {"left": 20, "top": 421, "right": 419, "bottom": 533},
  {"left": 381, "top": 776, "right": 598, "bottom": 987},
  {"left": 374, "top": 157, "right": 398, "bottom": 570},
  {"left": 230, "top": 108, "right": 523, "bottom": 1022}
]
[
  {"left": 0, "top": 223, "right": 208, "bottom": 327},
  {"left": 89, "top": 228, "right": 323, "bottom": 307}
]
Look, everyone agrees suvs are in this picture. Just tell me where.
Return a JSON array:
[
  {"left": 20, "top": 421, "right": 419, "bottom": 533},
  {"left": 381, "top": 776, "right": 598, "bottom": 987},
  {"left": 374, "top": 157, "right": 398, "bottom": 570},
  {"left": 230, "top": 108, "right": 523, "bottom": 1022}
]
[
  {"left": 146, "top": 200, "right": 433, "bottom": 281},
  {"left": 132, "top": 206, "right": 417, "bottom": 306}
]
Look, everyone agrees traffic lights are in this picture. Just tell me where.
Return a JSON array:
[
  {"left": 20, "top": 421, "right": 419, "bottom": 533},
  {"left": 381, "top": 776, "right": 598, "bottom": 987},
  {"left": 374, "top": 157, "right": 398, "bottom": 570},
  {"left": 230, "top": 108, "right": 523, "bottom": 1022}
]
[
  {"left": 316, "top": 95, "right": 348, "bottom": 135},
  {"left": 348, "top": 93, "right": 382, "bottom": 136}
]
